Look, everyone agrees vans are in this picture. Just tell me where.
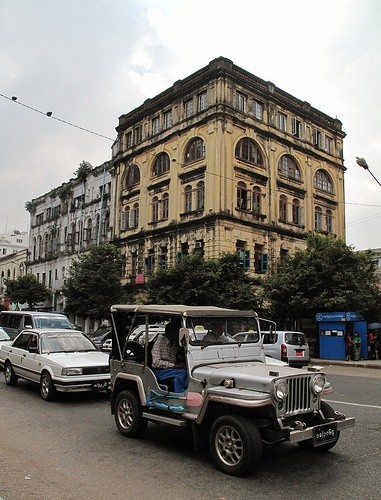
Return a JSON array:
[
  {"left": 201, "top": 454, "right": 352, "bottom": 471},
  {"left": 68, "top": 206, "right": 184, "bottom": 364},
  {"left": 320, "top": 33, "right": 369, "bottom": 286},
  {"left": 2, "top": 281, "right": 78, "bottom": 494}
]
[
  {"left": 230, "top": 330, "right": 312, "bottom": 368},
  {"left": 0, "top": 310, "right": 74, "bottom": 341}
]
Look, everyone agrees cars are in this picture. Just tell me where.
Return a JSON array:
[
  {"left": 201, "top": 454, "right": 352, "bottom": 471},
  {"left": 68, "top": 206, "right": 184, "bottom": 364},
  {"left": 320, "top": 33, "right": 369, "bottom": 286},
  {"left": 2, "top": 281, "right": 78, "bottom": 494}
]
[
  {"left": 106, "top": 303, "right": 357, "bottom": 479},
  {"left": 90, "top": 324, "right": 166, "bottom": 355},
  {"left": 0, "top": 327, "right": 110, "bottom": 402},
  {"left": 188, "top": 325, "right": 290, "bottom": 368}
]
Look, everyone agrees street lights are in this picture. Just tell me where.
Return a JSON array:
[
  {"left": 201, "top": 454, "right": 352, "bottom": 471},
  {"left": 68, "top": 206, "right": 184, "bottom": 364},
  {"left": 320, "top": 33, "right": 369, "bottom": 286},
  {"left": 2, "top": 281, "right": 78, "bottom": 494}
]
[{"left": 355, "top": 156, "right": 381, "bottom": 187}]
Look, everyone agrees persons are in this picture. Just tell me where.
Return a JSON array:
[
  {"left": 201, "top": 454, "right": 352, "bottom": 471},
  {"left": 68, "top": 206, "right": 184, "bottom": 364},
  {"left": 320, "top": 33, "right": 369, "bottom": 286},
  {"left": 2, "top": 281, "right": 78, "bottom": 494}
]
[
  {"left": 346, "top": 331, "right": 355, "bottom": 361},
  {"left": 369, "top": 329, "right": 376, "bottom": 360},
  {"left": 202, "top": 323, "right": 231, "bottom": 343},
  {"left": 151, "top": 323, "right": 189, "bottom": 393},
  {"left": 352, "top": 331, "right": 361, "bottom": 361}
]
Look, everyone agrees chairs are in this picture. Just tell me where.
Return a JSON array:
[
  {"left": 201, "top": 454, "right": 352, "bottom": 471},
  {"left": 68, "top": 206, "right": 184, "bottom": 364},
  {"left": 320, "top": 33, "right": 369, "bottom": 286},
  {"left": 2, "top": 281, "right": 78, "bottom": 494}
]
[{"left": 144, "top": 342, "right": 171, "bottom": 392}]
